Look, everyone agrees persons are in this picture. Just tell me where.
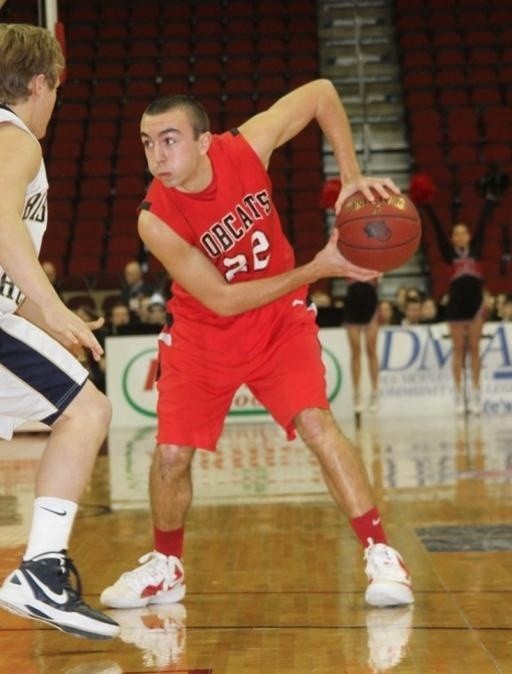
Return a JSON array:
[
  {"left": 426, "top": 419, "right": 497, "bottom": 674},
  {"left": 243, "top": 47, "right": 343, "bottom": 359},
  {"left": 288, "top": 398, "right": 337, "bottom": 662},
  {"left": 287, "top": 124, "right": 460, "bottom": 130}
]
[
  {"left": 102, "top": 602, "right": 421, "bottom": 673},
  {"left": 93, "top": 74, "right": 430, "bottom": 611},
  {"left": 344, "top": 245, "right": 386, "bottom": 423},
  {"left": 485, "top": 289, "right": 512, "bottom": 323},
  {"left": 43, "top": 259, "right": 173, "bottom": 396},
  {"left": 413, "top": 169, "right": 497, "bottom": 419},
  {"left": 0, "top": 20, "right": 128, "bottom": 645},
  {"left": 308, "top": 287, "right": 446, "bottom": 326}
]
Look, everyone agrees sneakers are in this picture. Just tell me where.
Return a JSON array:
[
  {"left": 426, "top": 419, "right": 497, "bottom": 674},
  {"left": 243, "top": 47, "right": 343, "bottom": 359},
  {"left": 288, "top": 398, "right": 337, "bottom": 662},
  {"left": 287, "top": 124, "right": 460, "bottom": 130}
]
[
  {"left": 0, "top": 547, "right": 122, "bottom": 640},
  {"left": 450, "top": 384, "right": 487, "bottom": 417},
  {"left": 351, "top": 384, "right": 384, "bottom": 419},
  {"left": 98, "top": 549, "right": 187, "bottom": 609},
  {"left": 361, "top": 539, "right": 416, "bottom": 609}
]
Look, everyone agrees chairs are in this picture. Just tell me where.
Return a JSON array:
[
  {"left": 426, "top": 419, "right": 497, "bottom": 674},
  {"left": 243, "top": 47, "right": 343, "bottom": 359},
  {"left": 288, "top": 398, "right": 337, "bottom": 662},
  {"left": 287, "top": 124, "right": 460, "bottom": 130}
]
[
  {"left": 389, "top": 0, "right": 512, "bottom": 296},
  {"left": 0, "top": 0, "right": 333, "bottom": 311}
]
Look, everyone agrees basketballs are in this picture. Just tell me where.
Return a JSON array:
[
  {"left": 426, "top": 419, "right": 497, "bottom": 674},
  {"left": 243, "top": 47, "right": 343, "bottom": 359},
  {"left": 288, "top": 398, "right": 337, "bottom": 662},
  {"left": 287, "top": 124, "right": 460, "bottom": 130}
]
[{"left": 335, "top": 184, "right": 422, "bottom": 271}]
